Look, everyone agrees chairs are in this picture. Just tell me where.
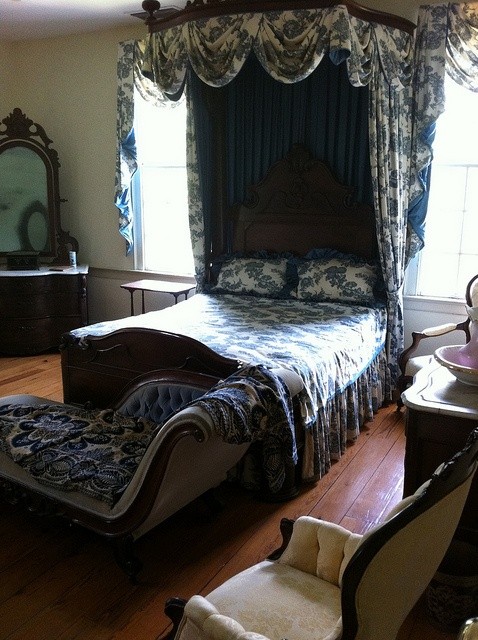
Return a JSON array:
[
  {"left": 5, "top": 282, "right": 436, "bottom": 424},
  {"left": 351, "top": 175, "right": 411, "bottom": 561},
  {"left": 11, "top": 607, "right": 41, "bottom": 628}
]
[
  {"left": 161, "top": 423, "right": 477, "bottom": 640},
  {"left": 394, "top": 274, "right": 478, "bottom": 414},
  {"left": 0, "top": 368, "right": 305, "bottom": 586}
]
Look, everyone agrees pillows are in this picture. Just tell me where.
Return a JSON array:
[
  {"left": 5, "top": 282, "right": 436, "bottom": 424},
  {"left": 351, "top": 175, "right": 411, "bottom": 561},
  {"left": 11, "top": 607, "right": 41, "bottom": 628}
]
[
  {"left": 295, "top": 247, "right": 380, "bottom": 311},
  {"left": 201, "top": 249, "right": 298, "bottom": 300}
]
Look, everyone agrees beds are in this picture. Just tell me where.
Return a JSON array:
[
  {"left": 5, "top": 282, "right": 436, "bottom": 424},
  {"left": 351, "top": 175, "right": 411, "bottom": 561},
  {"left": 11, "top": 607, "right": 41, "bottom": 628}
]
[{"left": 58, "top": 0, "right": 416, "bottom": 502}]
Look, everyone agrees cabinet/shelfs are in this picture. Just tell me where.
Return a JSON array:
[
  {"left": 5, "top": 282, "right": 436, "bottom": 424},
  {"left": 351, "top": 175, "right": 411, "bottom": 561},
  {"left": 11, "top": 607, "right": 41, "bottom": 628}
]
[
  {"left": 401, "top": 359, "right": 478, "bottom": 504},
  {"left": 0, "top": 277, "right": 88, "bottom": 358}
]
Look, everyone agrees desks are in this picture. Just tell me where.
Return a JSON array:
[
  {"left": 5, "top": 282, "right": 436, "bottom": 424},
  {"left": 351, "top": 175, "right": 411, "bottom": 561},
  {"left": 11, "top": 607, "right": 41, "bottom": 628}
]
[{"left": 119, "top": 278, "right": 198, "bottom": 317}]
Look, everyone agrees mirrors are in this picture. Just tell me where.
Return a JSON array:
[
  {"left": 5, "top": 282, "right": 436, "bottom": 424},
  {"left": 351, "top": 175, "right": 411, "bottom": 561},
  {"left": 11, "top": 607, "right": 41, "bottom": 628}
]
[{"left": 0, "top": 110, "right": 58, "bottom": 262}]
